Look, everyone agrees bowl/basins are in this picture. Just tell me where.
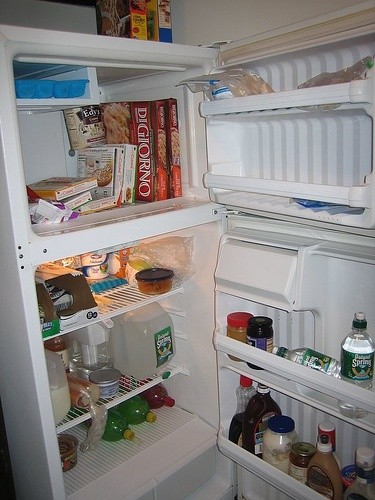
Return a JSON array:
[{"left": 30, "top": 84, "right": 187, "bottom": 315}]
[
  {"left": 55, "top": 433, "right": 81, "bottom": 473},
  {"left": 135, "top": 268, "right": 176, "bottom": 294}
]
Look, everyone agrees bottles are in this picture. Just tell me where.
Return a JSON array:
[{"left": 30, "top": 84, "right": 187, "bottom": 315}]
[
  {"left": 228, "top": 375, "right": 258, "bottom": 445},
  {"left": 306, "top": 422, "right": 344, "bottom": 500},
  {"left": 109, "top": 396, "right": 162, "bottom": 425},
  {"left": 341, "top": 312, "right": 375, "bottom": 418},
  {"left": 89, "top": 410, "right": 135, "bottom": 442},
  {"left": 344, "top": 446, "right": 374, "bottom": 500},
  {"left": 273, "top": 346, "right": 344, "bottom": 380},
  {"left": 142, "top": 383, "right": 181, "bottom": 408}
]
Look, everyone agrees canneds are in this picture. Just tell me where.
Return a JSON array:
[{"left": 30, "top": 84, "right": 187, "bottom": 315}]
[
  {"left": 226, "top": 312, "right": 255, "bottom": 362},
  {"left": 289, "top": 441, "right": 316, "bottom": 486},
  {"left": 88, "top": 368, "right": 121, "bottom": 399},
  {"left": 246, "top": 316, "right": 273, "bottom": 370}
]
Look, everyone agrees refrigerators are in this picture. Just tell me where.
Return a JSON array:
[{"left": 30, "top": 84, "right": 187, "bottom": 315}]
[{"left": 0, "top": 1, "right": 375, "bottom": 500}]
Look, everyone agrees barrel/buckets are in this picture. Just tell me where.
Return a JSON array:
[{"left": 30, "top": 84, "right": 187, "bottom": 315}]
[{"left": 109, "top": 303, "right": 176, "bottom": 380}]
